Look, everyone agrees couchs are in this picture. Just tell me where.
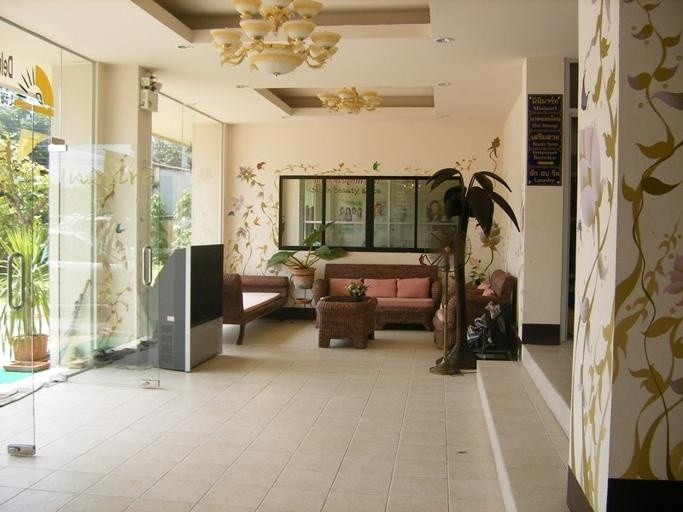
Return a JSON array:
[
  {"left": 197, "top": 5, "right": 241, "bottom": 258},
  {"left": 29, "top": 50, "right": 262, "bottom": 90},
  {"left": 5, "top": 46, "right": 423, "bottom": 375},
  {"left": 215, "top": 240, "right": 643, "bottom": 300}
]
[
  {"left": 312, "top": 264, "right": 439, "bottom": 331},
  {"left": 223, "top": 273, "right": 288, "bottom": 345},
  {"left": 434, "top": 268, "right": 518, "bottom": 350}
]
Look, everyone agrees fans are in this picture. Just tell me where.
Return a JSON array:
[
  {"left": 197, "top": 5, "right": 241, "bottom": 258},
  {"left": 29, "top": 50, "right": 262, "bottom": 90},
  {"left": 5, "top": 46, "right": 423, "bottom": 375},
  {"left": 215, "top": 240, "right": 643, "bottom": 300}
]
[{"left": 425, "top": 227, "right": 471, "bottom": 375}]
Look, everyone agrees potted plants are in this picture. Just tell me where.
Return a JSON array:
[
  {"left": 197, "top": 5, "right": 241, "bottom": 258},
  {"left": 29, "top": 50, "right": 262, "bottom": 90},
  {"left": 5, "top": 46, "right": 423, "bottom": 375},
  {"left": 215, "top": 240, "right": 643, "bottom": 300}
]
[
  {"left": 0, "top": 223, "right": 52, "bottom": 362},
  {"left": 266, "top": 220, "right": 347, "bottom": 289}
]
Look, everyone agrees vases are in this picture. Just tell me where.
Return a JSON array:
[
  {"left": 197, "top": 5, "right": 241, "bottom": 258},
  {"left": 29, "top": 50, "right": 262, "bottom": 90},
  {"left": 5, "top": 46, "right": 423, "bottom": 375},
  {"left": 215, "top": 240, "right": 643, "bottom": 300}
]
[{"left": 352, "top": 294, "right": 363, "bottom": 301}]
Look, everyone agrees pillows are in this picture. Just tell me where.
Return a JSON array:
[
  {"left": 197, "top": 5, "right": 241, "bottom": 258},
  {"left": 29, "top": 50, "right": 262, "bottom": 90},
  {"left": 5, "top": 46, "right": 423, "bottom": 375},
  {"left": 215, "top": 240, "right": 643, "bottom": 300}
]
[
  {"left": 477, "top": 282, "right": 491, "bottom": 289},
  {"left": 396, "top": 276, "right": 430, "bottom": 298},
  {"left": 482, "top": 289, "right": 496, "bottom": 296},
  {"left": 328, "top": 277, "right": 362, "bottom": 297},
  {"left": 364, "top": 279, "right": 397, "bottom": 298}
]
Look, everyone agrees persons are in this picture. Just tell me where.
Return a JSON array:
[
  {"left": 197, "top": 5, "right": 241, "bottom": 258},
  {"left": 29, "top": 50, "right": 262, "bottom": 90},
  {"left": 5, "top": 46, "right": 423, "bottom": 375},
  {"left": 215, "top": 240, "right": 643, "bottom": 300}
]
[
  {"left": 374, "top": 202, "right": 384, "bottom": 222},
  {"left": 338, "top": 205, "right": 364, "bottom": 227},
  {"left": 427, "top": 200, "right": 442, "bottom": 222}
]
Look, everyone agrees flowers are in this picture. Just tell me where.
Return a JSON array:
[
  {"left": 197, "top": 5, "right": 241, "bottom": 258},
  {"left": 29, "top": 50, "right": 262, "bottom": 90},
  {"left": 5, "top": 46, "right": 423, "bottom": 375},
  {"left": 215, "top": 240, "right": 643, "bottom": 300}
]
[{"left": 345, "top": 278, "right": 373, "bottom": 296}]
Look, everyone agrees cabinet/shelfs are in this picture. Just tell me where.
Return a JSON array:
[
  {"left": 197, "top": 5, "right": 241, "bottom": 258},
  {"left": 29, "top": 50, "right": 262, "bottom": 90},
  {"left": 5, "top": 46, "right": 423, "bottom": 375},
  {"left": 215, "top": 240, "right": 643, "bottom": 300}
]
[{"left": 289, "top": 274, "right": 315, "bottom": 324}]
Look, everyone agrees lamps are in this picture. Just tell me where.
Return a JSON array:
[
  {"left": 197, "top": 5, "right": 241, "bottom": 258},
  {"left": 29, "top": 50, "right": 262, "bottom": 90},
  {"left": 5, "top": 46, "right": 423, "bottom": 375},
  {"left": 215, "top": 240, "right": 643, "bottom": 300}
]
[
  {"left": 210, "top": 1, "right": 337, "bottom": 79},
  {"left": 318, "top": 87, "right": 383, "bottom": 113}
]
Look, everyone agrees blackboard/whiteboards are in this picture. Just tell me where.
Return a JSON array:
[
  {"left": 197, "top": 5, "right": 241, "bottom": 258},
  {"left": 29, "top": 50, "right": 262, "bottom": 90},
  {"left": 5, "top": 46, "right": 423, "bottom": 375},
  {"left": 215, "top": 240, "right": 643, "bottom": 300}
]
[{"left": 525, "top": 93, "right": 563, "bottom": 187}]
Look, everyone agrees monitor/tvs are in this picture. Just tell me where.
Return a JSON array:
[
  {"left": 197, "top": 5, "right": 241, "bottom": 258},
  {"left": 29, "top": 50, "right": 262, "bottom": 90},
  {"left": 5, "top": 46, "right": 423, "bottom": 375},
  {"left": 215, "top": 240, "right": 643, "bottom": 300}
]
[{"left": 149, "top": 243, "right": 224, "bottom": 372}]
